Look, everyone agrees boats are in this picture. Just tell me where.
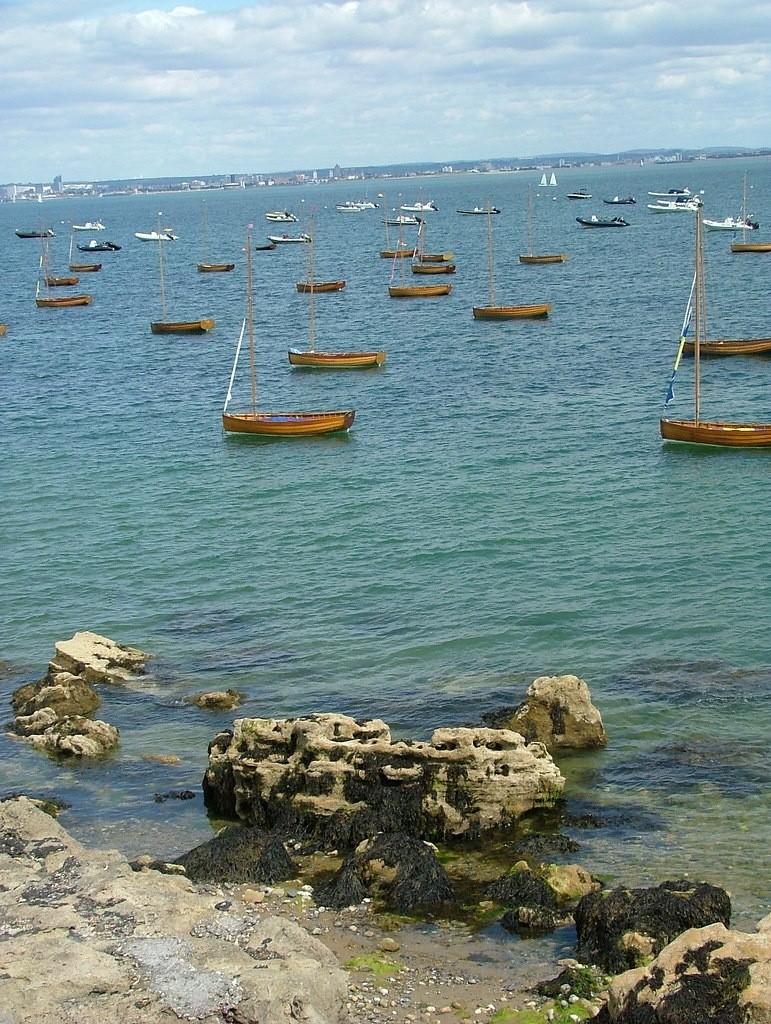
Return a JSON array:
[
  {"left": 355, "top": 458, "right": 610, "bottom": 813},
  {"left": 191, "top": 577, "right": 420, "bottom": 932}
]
[
  {"left": 296, "top": 280, "right": 345, "bottom": 293},
  {"left": 73, "top": 221, "right": 105, "bottom": 231},
  {"left": 77, "top": 238, "right": 122, "bottom": 251},
  {"left": 36, "top": 217, "right": 102, "bottom": 308},
  {"left": 519, "top": 182, "right": 567, "bottom": 264},
  {"left": 472, "top": 196, "right": 552, "bottom": 319},
  {"left": 197, "top": 262, "right": 235, "bottom": 272},
  {"left": 0, "top": 324, "right": 7, "bottom": 336},
  {"left": 380, "top": 186, "right": 506, "bottom": 296},
  {"left": 150, "top": 212, "right": 214, "bottom": 332},
  {"left": 15, "top": 230, "right": 57, "bottom": 238},
  {"left": 255, "top": 243, "right": 276, "bottom": 250},
  {"left": 336, "top": 201, "right": 380, "bottom": 213},
  {"left": 265, "top": 210, "right": 299, "bottom": 222},
  {"left": 288, "top": 204, "right": 386, "bottom": 369},
  {"left": 134, "top": 229, "right": 179, "bottom": 242},
  {"left": 660, "top": 198, "right": 771, "bottom": 448},
  {"left": 538, "top": 170, "right": 771, "bottom": 253},
  {"left": 267, "top": 234, "right": 314, "bottom": 244},
  {"left": 223, "top": 224, "right": 357, "bottom": 435}
]
